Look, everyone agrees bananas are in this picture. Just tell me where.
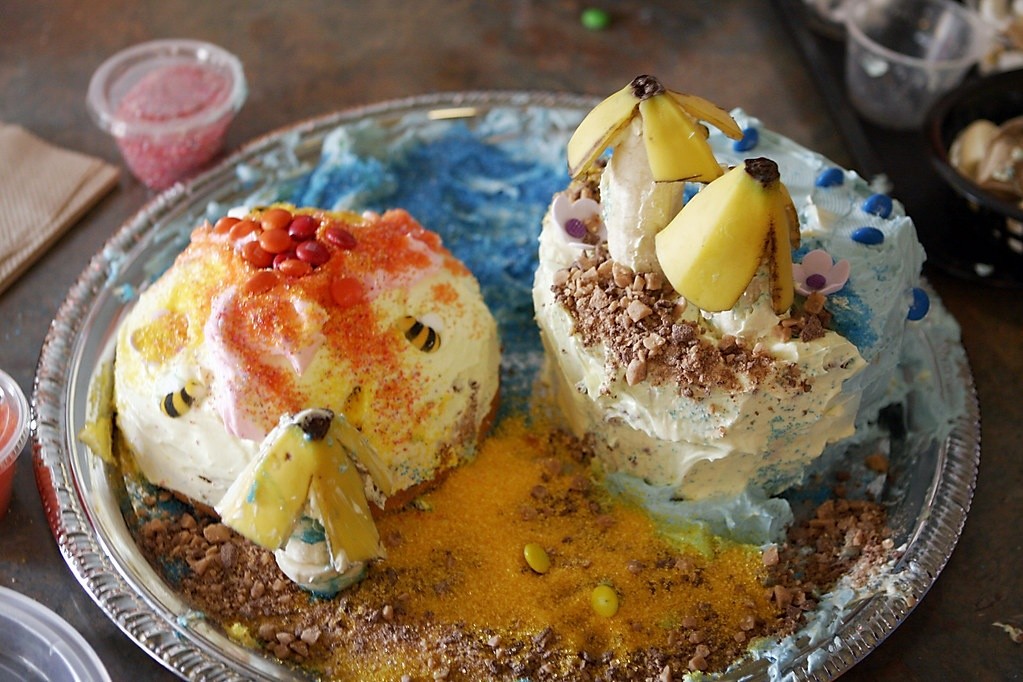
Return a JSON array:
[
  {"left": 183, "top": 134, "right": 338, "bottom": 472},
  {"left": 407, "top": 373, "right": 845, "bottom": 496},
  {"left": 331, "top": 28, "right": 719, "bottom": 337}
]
[{"left": 210, "top": 408, "right": 400, "bottom": 597}]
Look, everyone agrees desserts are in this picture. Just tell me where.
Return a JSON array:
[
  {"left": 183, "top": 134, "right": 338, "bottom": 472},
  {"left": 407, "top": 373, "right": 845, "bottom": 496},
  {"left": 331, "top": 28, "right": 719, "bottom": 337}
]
[
  {"left": 105, "top": 199, "right": 504, "bottom": 524},
  {"left": 529, "top": 75, "right": 925, "bottom": 545}
]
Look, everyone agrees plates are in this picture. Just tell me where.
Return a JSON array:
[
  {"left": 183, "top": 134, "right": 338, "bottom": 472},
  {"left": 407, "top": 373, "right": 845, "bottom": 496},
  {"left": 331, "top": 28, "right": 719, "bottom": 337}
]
[{"left": 29, "top": 89, "right": 982, "bottom": 682}]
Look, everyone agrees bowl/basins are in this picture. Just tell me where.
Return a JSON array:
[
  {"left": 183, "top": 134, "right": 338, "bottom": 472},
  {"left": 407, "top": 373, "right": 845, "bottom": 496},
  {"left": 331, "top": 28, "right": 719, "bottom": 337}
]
[
  {"left": 807, "top": 0, "right": 1023, "bottom": 332},
  {"left": 0, "top": 585, "right": 113, "bottom": 682},
  {"left": 0, "top": 368, "right": 32, "bottom": 517},
  {"left": 83, "top": 38, "right": 249, "bottom": 192}
]
